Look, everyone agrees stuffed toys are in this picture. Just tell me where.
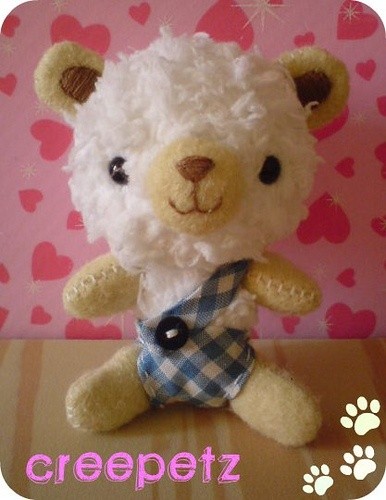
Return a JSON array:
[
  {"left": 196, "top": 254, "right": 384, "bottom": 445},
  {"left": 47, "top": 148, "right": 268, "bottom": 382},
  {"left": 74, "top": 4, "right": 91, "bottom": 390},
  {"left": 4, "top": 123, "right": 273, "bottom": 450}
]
[{"left": 33, "top": 28, "right": 352, "bottom": 445}]
